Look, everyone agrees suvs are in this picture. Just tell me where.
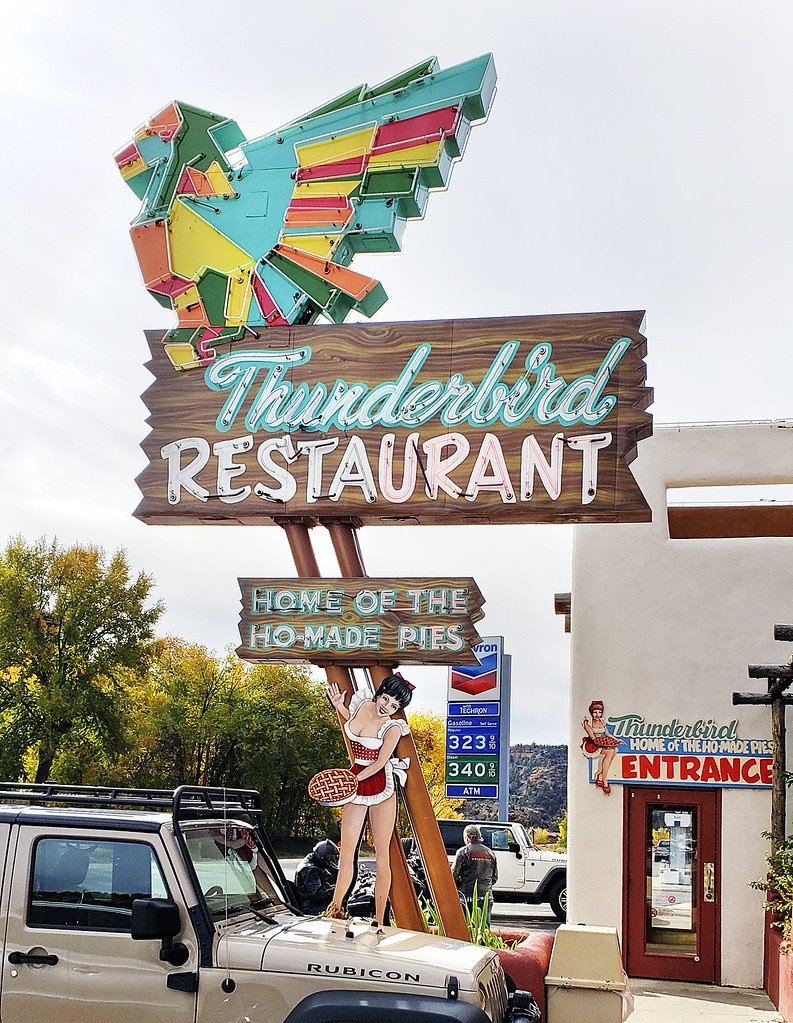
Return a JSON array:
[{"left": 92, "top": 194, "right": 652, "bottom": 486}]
[
  {"left": 401, "top": 818, "right": 568, "bottom": 921},
  {"left": 1, "top": 780, "right": 543, "bottom": 1023},
  {"left": 654, "top": 839, "right": 670, "bottom": 862}
]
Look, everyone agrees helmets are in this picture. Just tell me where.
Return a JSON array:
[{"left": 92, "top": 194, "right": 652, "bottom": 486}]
[{"left": 311, "top": 838, "right": 339, "bottom": 869}]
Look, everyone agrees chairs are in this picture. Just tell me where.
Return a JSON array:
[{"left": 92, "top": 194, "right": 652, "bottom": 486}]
[{"left": 42, "top": 851, "right": 105, "bottom": 929}]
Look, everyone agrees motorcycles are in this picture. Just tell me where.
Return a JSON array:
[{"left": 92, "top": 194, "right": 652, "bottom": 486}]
[
  {"left": 279, "top": 863, "right": 380, "bottom": 918},
  {"left": 399, "top": 862, "right": 439, "bottom": 926}
]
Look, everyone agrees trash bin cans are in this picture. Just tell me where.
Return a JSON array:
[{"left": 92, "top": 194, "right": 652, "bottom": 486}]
[{"left": 544, "top": 921, "right": 635, "bottom": 1023}]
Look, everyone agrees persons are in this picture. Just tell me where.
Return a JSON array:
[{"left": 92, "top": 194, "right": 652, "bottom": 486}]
[
  {"left": 452, "top": 823, "right": 499, "bottom": 931},
  {"left": 292, "top": 839, "right": 341, "bottom": 915}
]
